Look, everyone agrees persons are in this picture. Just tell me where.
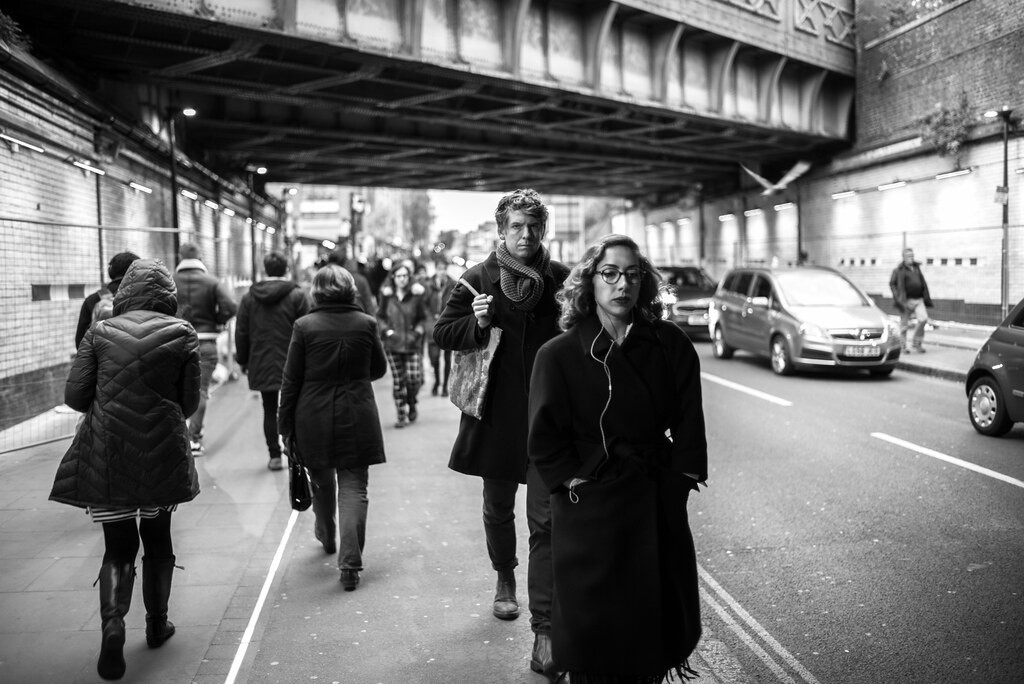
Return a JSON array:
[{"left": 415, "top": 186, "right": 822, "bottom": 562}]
[
  {"left": 315, "top": 249, "right": 458, "bottom": 428},
  {"left": 235, "top": 252, "right": 308, "bottom": 471},
  {"left": 172, "top": 243, "right": 235, "bottom": 456},
  {"left": 434, "top": 188, "right": 570, "bottom": 675},
  {"left": 526, "top": 233, "right": 708, "bottom": 684},
  {"left": 48, "top": 258, "right": 202, "bottom": 681},
  {"left": 75, "top": 252, "right": 140, "bottom": 349},
  {"left": 277, "top": 263, "right": 388, "bottom": 590},
  {"left": 890, "top": 249, "right": 933, "bottom": 355}
]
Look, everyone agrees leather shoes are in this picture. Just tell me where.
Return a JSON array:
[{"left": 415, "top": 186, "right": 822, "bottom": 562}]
[
  {"left": 493, "top": 571, "right": 520, "bottom": 618},
  {"left": 531, "top": 633, "right": 554, "bottom": 671}
]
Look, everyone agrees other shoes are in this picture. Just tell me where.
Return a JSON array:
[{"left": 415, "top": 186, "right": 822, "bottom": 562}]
[
  {"left": 268, "top": 457, "right": 283, "bottom": 471},
  {"left": 408, "top": 404, "right": 417, "bottom": 422},
  {"left": 431, "top": 381, "right": 439, "bottom": 396},
  {"left": 340, "top": 569, "right": 360, "bottom": 591},
  {"left": 315, "top": 529, "right": 337, "bottom": 554},
  {"left": 901, "top": 349, "right": 909, "bottom": 353},
  {"left": 912, "top": 340, "right": 925, "bottom": 352},
  {"left": 189, "top": 440, "right": 204, "bottom": 457},
  {"left": 395, "top": 421, "right": 405, "bottom": 427},
  {"left": 442, "top": 385, "right": 448, "bottom": 397}
]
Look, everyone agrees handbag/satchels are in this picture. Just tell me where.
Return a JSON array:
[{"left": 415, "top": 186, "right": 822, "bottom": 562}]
[
  {"left": 285, "top": 437, "right": 312, "bottom": 512},
  {"left": 450, "top": 279, "right": 502, "bottom": 420}
]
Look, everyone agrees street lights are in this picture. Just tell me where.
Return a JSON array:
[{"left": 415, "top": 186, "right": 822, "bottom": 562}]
[
  {"left": 282, "top": 187, "right": 298, "bottom": 273},
  {"left": 168, "top": 106, "right": 197, "bottom": 269},
  {"left": 983, "top": 105, "right": 1013, "bottom": 324},
  {"left": 248, "top": 164, "right": 268, "bottom": 288}
]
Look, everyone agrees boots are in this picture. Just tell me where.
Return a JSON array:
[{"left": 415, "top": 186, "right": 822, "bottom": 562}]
[
  {"left": 93, "top": 562, "right": 136, "bottom": 682},
  {"left": 141, "top": 554, "right": 185, "bottom": 648}
]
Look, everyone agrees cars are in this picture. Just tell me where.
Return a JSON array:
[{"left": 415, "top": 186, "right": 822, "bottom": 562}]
[{"left": 651, "top": 266, "right": 720, "bottom": 341}]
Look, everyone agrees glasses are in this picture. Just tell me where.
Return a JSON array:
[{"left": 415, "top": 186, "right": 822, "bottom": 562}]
[
  {"left": 394, "top": 274, "right": 408, "bottom": 279},
  {"left": 594, "top": 268, "right": 646, "bottom": 285}
]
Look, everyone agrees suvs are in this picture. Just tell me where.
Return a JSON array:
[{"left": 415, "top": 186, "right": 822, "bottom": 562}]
[{"left": 706, "top": 261, "right": 904, "bottom": 378}]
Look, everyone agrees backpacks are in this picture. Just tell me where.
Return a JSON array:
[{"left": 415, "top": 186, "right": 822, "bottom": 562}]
[{"left": 91, "top": 286, "right": 115, "bottom": 323}]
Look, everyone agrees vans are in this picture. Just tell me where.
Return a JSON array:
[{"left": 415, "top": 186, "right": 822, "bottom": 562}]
[{"left": 965, "top": 298, "right": 1024, "bottom": 438}]
